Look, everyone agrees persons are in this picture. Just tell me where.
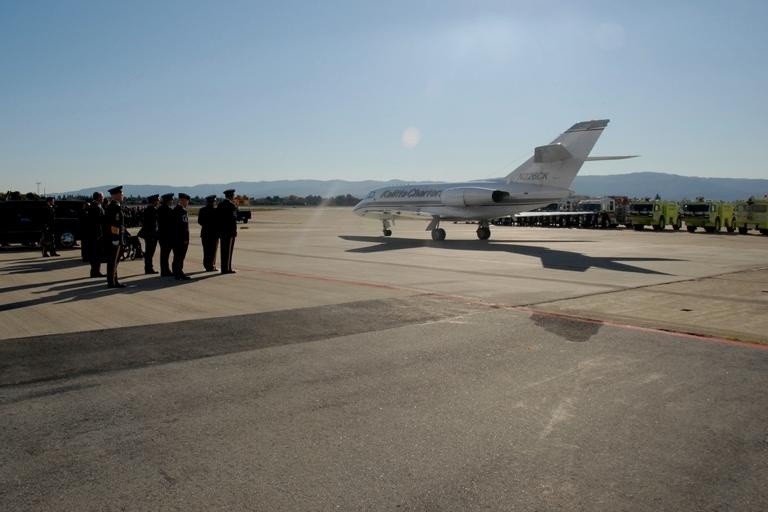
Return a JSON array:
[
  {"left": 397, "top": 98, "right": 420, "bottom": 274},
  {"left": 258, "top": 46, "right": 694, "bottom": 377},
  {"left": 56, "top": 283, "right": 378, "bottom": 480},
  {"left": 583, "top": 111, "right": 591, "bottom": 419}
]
[
  {"left": 105, "top": 185, "right": 126, "bottom": 288},
  {"left": 87, "top": 191, "right": 103, "bottom": 280},
  {"left": 156, "top": 194, "right": 174, "bottom": 277},
  {"left": 492, "top": 205, "right": 612, "bottom": 230},
  {"left": 196, "top": 193, "right": 219, "bottom": 271},
  {"left": 40, "top": 194, "right": 63, "bottom": 257},
  {"left": 173, "top": 192, "right": 192, "bottom": 280},
  {"left": 215, "top": 189, "right": 241, "bottom": 274},
  {"left": 137, "top": 192, "right": 158, "bottom": 276},
  {"left": 102, "top": 198, "right": 145, "bottom": 229}
]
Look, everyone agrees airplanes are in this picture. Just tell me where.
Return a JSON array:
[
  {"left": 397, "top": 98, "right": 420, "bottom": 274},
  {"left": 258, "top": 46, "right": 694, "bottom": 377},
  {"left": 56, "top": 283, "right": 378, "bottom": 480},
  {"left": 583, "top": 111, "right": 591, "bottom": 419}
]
[{"left": 351, "top": 118, "right": 640, "bottom": 244}]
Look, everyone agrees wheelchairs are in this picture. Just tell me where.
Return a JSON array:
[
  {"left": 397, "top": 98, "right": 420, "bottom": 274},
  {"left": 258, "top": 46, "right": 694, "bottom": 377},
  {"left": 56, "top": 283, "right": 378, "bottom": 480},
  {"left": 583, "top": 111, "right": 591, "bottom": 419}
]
[{"left": 120, "top": 235, "right": 144, "bottom": 260}]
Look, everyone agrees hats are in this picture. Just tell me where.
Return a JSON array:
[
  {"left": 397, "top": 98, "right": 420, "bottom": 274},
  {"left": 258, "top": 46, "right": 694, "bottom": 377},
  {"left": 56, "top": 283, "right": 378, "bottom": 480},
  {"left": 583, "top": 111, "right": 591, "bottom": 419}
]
[
  {"left": 205, "top": 194, "right": 217, "bottom": 202},
  {"left": 178, "top": 193, "right": 191, "bottom": 201},
  {"left": 159, "top": 193, "right": 175, "bottom": 200},
  {"left": 222, "top": 188, "right": 236, "bottom": 197},
  {"left": 107, "top": 185, "right": 124, "bottom": 195},
  {"left": 146, "top": 194, "right": 160, "bottom": 201}
]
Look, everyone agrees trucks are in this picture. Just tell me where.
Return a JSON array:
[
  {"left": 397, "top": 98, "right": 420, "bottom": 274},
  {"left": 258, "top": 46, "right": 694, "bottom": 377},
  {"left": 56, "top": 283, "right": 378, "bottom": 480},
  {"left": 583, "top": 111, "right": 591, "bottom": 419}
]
[{"left": 538, "top": 197, "right": 768, "bottom": 235}]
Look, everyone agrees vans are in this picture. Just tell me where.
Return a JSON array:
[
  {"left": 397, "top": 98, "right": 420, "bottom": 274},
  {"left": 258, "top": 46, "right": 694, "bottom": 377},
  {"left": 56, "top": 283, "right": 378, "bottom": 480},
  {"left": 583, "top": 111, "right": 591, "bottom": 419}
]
[{"left": 0, "top": 198, "right": 88, "bottom": 250}]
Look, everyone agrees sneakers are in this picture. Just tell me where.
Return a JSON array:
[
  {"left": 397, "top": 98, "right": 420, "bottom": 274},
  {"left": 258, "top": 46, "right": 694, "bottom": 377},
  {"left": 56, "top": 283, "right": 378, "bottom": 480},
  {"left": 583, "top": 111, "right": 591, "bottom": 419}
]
[{"left": 205, "top": 268, "right": 238, "bottom": 273}]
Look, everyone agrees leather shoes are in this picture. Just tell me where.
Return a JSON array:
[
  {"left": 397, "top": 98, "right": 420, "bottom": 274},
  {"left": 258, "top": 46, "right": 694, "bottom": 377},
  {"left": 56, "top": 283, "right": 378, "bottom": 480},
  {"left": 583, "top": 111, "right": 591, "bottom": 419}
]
[
  {"left": 89, "top": 272, "right": 108, "bottom": 278},
  {"left": 143, "top": 271, "right": 192, "bottom": 281},
  {"left": 42, "top": 251, "right": 62, "bottom": 258},
  {"left": 105, "top": 283, "right": 126, "bottom": 288}
]
